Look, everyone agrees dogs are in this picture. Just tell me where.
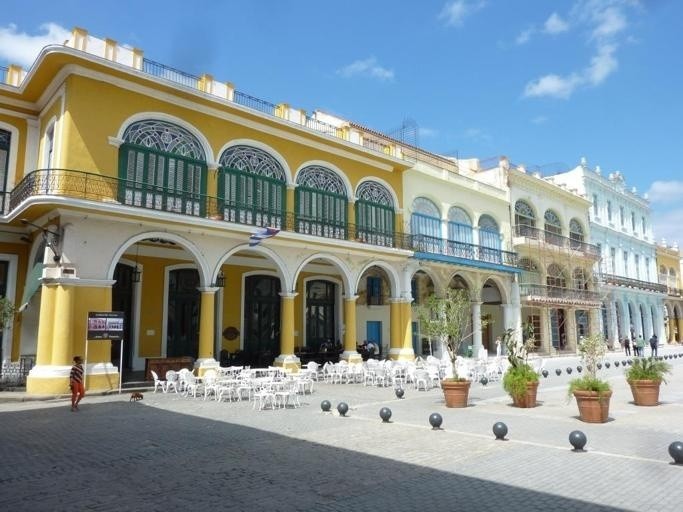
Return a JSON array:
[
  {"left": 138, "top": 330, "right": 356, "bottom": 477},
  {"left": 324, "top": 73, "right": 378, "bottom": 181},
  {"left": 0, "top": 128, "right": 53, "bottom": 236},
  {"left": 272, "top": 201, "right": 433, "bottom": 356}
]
[{"left": 129, "top": 392, "right": 144, "bottom": 402}]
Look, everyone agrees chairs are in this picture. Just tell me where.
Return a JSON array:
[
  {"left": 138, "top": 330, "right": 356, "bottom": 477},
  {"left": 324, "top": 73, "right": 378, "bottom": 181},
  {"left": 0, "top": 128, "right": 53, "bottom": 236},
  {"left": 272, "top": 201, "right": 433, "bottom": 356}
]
[{"left": 150, "top": 356, "right": 413, "bottom": 413}]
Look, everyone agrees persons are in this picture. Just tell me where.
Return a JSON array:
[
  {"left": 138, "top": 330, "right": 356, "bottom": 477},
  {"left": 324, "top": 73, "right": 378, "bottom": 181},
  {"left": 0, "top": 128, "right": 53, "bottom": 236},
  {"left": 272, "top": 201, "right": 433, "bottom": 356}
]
[
  {"left": 70, "top": 356, "right": 84, "bottom": 411},
  {"left": 650, "top": 334, "right": 658, "bottom": 358},
  {"left": 367, "top": 340, "right": 380, "bottom": 355},
  {"left": 624, "top": 335, "right": 645, "bottom": 357}
]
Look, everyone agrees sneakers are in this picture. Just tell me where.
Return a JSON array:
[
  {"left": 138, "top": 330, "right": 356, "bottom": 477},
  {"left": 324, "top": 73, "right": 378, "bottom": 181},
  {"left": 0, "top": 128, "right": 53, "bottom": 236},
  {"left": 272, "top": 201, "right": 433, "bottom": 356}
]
[{"left": 72, "top": 404, "right": 80, "bottom": 412}]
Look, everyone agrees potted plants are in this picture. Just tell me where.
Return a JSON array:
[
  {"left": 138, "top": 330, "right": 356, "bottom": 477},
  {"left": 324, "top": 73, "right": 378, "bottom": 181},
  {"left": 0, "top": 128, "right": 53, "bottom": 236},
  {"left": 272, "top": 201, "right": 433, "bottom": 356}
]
[{"left": 414, "top": 283, "right": 674, "bottom": 423}]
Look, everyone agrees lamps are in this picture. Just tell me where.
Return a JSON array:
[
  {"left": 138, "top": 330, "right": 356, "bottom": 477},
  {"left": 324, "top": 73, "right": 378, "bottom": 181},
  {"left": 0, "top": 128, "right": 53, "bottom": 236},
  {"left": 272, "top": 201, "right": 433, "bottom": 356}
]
[
  {"left": 217, "top": 266, "right": 225, "bottom": 287},
  {"left": 130, "top": 245, "right": 141, "bottom": 282}
]
